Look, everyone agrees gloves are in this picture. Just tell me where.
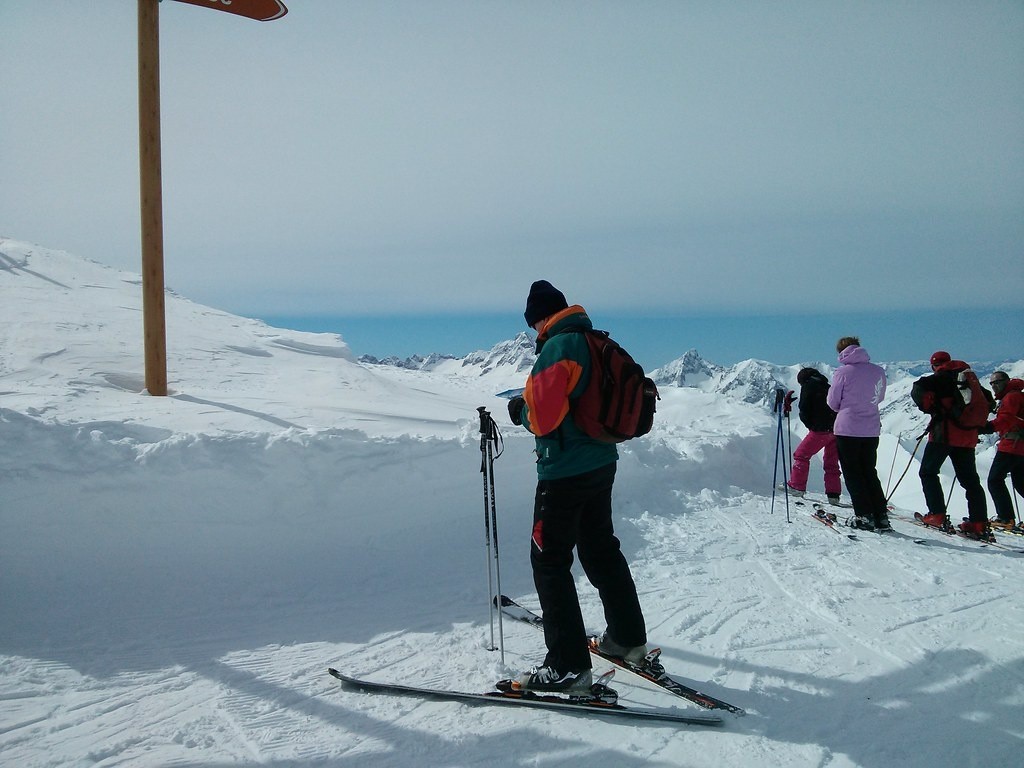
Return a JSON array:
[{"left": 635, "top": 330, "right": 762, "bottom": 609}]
[
  {"left": 783, "top": 390, "right": 798, "bottom": 411},
  {"left": 508, "top": 397, "right": 526, "bottom": 425}
]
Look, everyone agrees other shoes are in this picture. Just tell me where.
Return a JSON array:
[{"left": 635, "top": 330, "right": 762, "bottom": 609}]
[
  {"left": 990, "top": 518, "right": 1015, "bottom": 530},
  {"left": 961, "top": 521, "right": 985, "bottom": 535},
  {"left": 778, "top": 482, "right": 804, "bottom": 496},
  {"left": 828, "top": 496, "right": 840, "bottom": 505},
  {"left": 922, "top": 511, "right": 947, "bottom": 530},
  {"left": 877, "top": 518, "right": 893, "bottom": 531},
  {"left": 847, "top": 516, "right": 875, "bottom": 530}
]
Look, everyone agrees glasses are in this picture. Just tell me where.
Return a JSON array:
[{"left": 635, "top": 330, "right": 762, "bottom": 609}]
[
  {"left": 990, "top": 379, "right": 1005, "bottom": 385},
  {"left": 932, "top": 364, "right": 939, "bottom": 372}
]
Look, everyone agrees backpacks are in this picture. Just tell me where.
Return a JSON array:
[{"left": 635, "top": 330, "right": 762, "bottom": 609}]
[
  {"left": 938, "top": 362, "right": 990, "bottom": 430},
  {"left": 551, "top": 325, "right": 661, "bottom": 441}
]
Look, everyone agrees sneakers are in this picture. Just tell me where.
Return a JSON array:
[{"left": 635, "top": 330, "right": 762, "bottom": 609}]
[
  {"left": 594, "top": 631, "right": 648, "bottom": 667},
  {"left": 519, "top": 665, "right": 592, "bottom": 701}
]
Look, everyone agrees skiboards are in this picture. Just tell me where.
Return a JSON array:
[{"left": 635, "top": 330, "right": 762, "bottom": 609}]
[
  {"left": 494, "top": 593, "right": 747, "bottom": 717},
  {"left": 327, "top": 665, "right": 725, "bottom": 727},
  {"left": 812, "top": 503, "right": 927, "bottom": 544},
  {"left": 961, "top": 516, "right": 1024, "bottom": 536},
  {"left": 913, "top": 512, "right": 1024, "bottom": 553}
]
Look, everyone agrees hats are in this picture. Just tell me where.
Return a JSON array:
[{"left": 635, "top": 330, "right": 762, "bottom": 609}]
[
  {"left": 930, "top": 352, "right": 950, "bottom": 365},
  {"left": 524, "top": 280, "right": 568, "bottom": 327}
]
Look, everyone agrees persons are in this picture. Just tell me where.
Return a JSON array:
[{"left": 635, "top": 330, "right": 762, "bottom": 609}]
[
  {"left": 910, "top": 351, "right": 993, "bottom": 535},
  {"left": 827, "top": 336, "right": 895, "bottom": 534},
  {"left": 778, "top": 367, "right": 842, "bottom": 505},
  {"left": 496, "top": 280, "right": 662, "bottom": 694},
  {"left": 978, "top": 371, "right": 1024, "bottom": 528}
]
